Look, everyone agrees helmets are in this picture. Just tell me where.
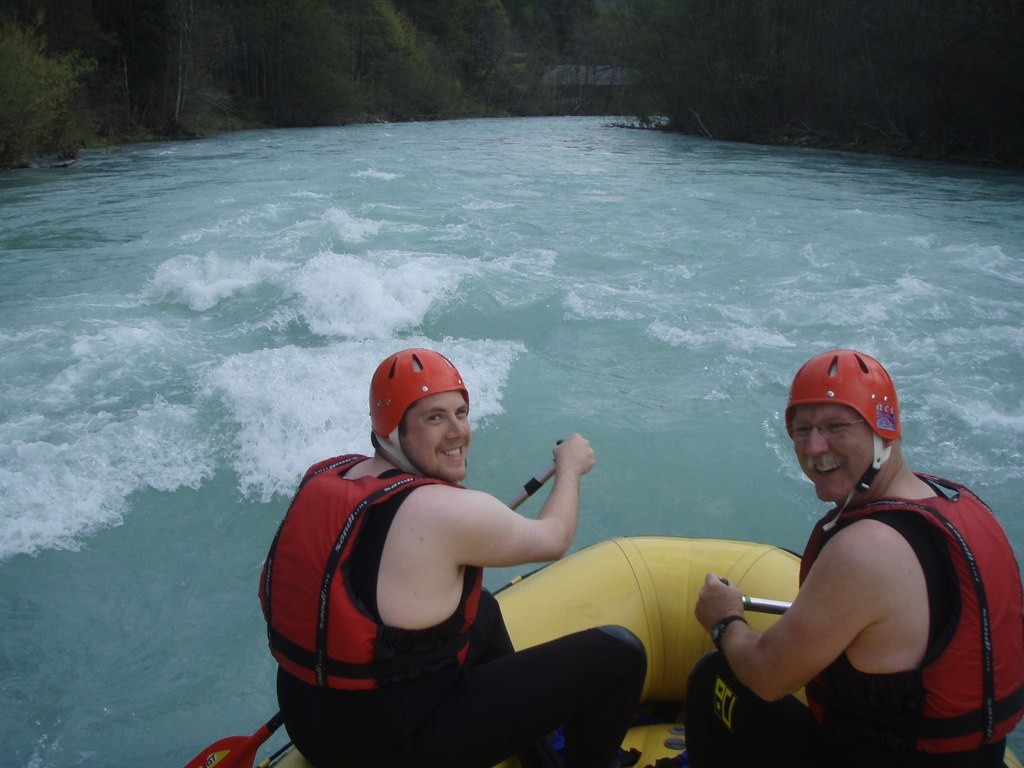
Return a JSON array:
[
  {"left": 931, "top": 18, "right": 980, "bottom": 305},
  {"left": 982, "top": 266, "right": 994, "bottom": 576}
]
[
  {"left": 369, "top": 348, "right": 467, "bottom": 439},
  {"left": 785, "top": 349, "right": 901, "bottom": 440}
]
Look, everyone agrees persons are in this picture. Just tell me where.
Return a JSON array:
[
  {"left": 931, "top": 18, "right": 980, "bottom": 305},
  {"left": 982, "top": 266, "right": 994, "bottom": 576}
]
[
  {"left": 682, "top": 347, "right": 1024, "bottom": 768},
  {"left": 257, "top": 346, "right": 647, "bottom": 768}
]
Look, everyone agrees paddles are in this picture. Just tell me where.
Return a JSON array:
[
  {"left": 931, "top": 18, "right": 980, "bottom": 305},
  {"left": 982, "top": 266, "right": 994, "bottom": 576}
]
[
  {"left": 718, "top": 577, "right": 793, "bottom": 614},
  {"left": 182, "top": 436, "right": 568, "bottom": 768}
]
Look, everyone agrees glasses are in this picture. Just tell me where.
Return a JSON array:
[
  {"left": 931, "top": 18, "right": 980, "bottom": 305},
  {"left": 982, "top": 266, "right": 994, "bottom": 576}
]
[{"left": 788, "top": 416, "right": 868, "bottom": 439}]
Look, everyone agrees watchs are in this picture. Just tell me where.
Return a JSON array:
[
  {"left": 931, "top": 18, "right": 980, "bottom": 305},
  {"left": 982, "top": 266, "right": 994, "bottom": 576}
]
[{"left": 711, "top": 614, "right": 749, "bottom": 653}]
